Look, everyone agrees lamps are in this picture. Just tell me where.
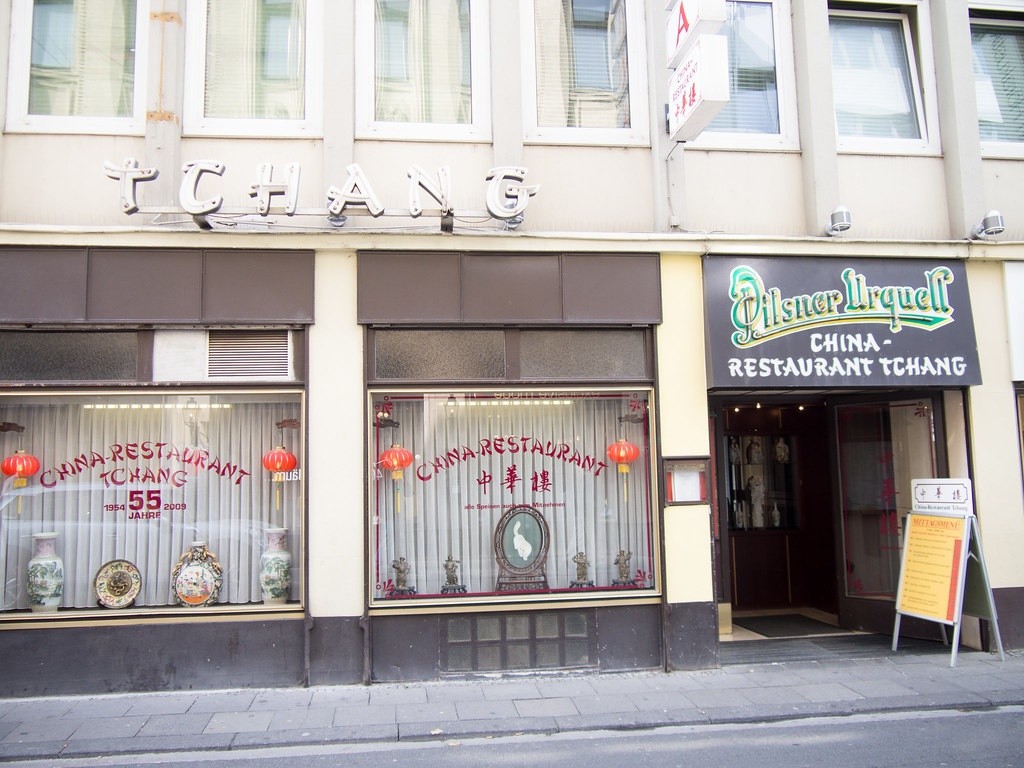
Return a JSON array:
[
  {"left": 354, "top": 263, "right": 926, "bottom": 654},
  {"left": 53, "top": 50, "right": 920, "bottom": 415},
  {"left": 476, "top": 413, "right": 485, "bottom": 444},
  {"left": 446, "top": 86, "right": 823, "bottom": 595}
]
[
  {"left": 972, "top": 215, "right": 1004, "bottom": 240},
  {"left": 824, "top": 211, "right": 852, "bottom": 236}
]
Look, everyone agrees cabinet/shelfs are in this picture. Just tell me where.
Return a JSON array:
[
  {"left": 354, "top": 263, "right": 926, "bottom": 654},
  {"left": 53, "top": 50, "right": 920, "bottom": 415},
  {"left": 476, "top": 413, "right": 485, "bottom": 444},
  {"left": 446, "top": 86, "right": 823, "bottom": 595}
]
[{"left": 722, "top": 430, "right": 800, "bottom": 538}]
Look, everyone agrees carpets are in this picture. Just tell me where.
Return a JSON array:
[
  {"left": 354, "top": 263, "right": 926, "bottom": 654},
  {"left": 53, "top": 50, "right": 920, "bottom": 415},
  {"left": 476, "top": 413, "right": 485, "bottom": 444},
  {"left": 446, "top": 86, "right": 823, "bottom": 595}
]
[{"left": 732, "top": 613, "right": 856, "bottom": 638}]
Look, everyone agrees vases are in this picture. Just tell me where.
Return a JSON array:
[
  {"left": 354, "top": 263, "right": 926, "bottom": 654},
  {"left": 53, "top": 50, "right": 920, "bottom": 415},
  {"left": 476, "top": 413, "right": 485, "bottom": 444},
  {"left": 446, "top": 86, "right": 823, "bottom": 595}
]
[
  {"left": 259, "top": 527, "right": 293, "bottom": 604},
  {"left": 27, "top": 532, "right": 64, "bottom": 613},
  {"left": 170, "top": 541, "right": 224, "bottom": 609}
]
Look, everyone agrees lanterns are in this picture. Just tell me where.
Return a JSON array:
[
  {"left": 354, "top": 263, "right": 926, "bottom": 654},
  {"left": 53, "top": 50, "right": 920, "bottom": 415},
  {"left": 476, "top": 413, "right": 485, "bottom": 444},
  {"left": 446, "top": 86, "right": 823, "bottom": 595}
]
[
  {"left": 606, "top": 438, "right": 639, "bottom": 503},
  {"left": 262, "top": 445, "right": 297, "bottom": 511},
  {"left": 380, "top": 444, "right": 414, "bottom": 513},
  {"left": 1, "top": 450, "right": 40, "bottom": 514}
]
